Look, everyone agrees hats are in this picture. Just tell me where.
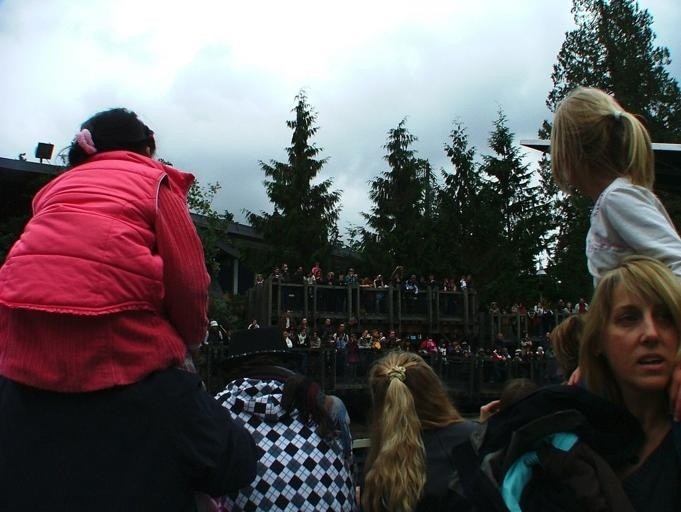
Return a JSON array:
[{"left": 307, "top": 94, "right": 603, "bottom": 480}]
[{"left": 223, "top": 325, "right": 307, "bottom": 360}]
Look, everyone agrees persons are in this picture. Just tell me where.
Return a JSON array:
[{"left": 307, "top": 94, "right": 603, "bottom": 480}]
[
  {"left": 2, "top": 366, "right": 258, "bottom": 511},
  {"left": 197, "top": 260, "right": 589, "bottom": 389},
  {"left": 474, "top": 376, "right": 535, "bottom": 422},
  {"left": 0, "top": 104, "right": 211, "bottom": 390},
  {"left": 551, "top": 313, "right": 593, "bottom": 371},
  {"left": 549, "top": 88, "right": 681, "bottom": 426},
  {"left": 357, "top": 348, "right": 492, "bottom": 510},
  {"left": 578, "top": 254, "right": 681, "bottom": 510},
  {"left": 210, "top": 323, "right": 360, "bottom": 512}
]
